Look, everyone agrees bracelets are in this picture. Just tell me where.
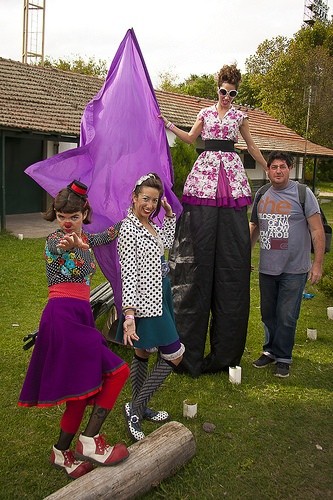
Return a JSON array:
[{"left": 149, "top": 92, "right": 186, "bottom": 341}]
[
  {"left": 165, "top": 214, "right": 175, "bottom": 218},
  {"left": 125, "top": 315, "right": 134, "bottom": 319},
  {"left": 169, "top": 124, "right": 174, "bottom": 130},
  {"left": 166, "top": 121, "right": 172, "bottom": 128}
]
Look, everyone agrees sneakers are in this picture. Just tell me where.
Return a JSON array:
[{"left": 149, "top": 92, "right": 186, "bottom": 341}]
[
  {"left": 48, "top": 444, "right": 92, "bottom": 479},
  {"left": 75, "top": 430, "right": 129, "bottom": 466},
  {"left": 273, "top": 362, "right": 291, "bottom": 377},
  {"left": 251, "top": 354, "right": 275, "bottom": 368}
]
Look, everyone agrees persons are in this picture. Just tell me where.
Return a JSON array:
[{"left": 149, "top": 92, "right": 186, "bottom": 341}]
[
  {"left": 18, "top": 180, "right": 130, "bottom": 480},
  {"left": 157, "top": 65, "right": 268, "bottom": 378},
  {"left": 249, "top": 151, "right": 325, "bottom": 378},
  {"left": 116, "top": 173, "right": 186, "bottom": 441}
]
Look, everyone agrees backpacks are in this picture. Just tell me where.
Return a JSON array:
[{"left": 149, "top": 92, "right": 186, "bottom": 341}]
[{"left": 252, "top": 182, "right": 332, "bottom": 254}]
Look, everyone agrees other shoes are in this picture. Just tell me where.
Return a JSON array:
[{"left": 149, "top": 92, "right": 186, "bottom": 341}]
[
  {"left": 143, "top": 410, "right": 169, "bottom": 422},
  {"left": 122, "top": 402, "right": 145, "bottom": 441}
]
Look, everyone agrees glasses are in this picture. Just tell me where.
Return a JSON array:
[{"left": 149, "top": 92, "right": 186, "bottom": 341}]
[{"left": 217, "top": 89, "right": 238, "bottom": 98}]
